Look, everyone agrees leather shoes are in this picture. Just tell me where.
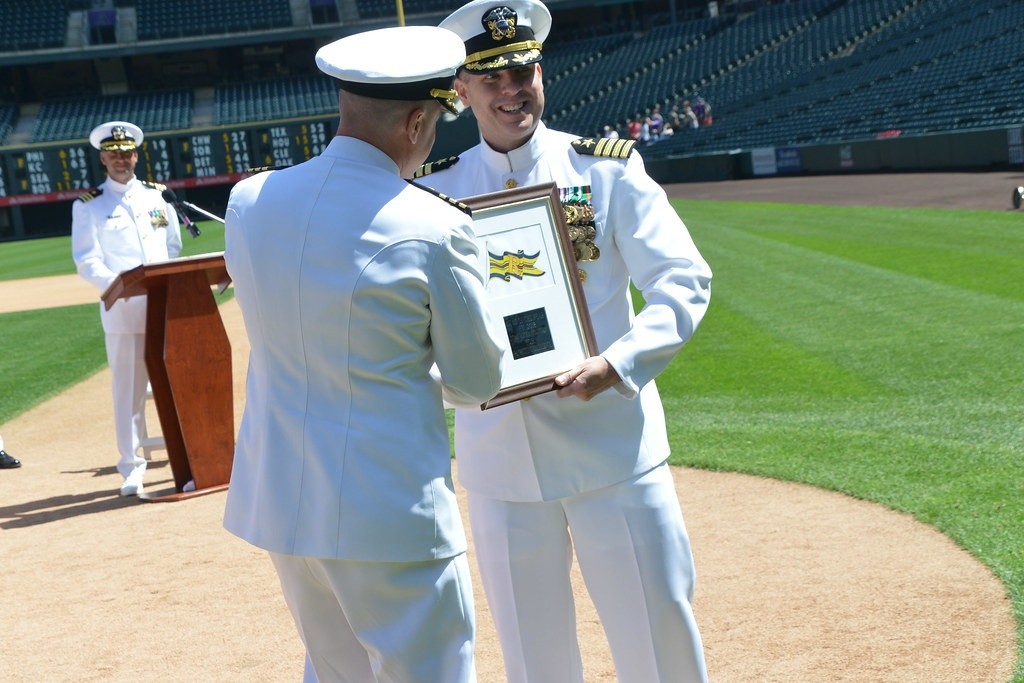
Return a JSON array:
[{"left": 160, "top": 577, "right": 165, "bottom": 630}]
[
  {"left": 0, "top": 450, "right": 20, "bottom": 468},
  {"left": 119, "top": 474, "right": 143, "bottom": 497}
]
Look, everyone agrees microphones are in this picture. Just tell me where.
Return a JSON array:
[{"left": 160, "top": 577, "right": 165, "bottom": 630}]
[{"left": 162, "top": 189, "right": 201, "bottom": 240}]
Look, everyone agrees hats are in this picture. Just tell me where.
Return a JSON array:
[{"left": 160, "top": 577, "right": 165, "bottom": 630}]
[
  {"left": 90, "top": 121, "right": 144, "bottom": 153},
  {"left": 433, "top": 0, "right": 552, "bottom": 78},
  {"left": 315, "top": 26, "right": 467, "bottom": 118}
]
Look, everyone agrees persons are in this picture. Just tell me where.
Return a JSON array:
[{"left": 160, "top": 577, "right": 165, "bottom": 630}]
[
  {"left": 407, "top": 0, "right": 716, "bottom": 682},
  {"left": 70, "top": 121, "right": 184, "bottom": 494},
  {"left": 589, "top": 94, "right": 713, "bottom": 155},
  {"left": 216, "top": 25, "right": 502, "bottom": 683}
]
